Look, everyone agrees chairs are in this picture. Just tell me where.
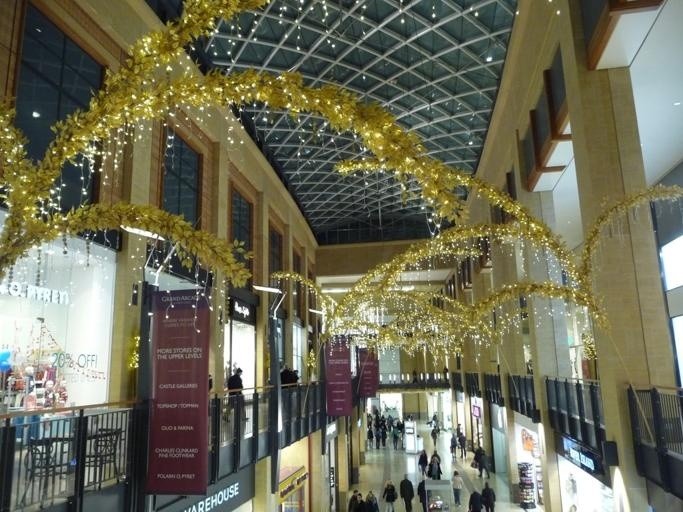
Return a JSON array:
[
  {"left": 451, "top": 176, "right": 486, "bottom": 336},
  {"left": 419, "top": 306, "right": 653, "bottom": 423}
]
[
  {"left": 16, "top": 437, "right": 68, "bottom": 511},
  {"left": 80, "top": 426, "right": 124, "bottom": 493}
]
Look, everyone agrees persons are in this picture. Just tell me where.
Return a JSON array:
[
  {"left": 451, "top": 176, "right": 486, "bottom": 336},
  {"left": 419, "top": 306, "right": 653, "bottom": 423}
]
[
  {"left": 413, "top": 368, "right": 419, "bottom": 384},
  {"left": 45, "top": 381, "right": 56, "bottom": 407},
  {"left": 348, "top": 490, "right": 379, "bottom": 512},
  {"left": 280, "top": 365, "right": 299, "bottom": 388},
  {"left": 431, "top": 415, "right": 439, "bottom": 446},
  {"left": 366, "top": 408, "right": 404, "bottom": 450},
  {"left": 451, "top": 432, "right": 490, "bottom": 479},
  {"left": 469, "top": 482, "right": 496, "bottom": 512},
  {"left": 382, "top": 474, "right": 413, "bottom": 512},
  {"left": 25, "top": 367, "right": 35, "bottom": 394},
  {"left": 451, "top": 471, "right": 463, "bottom": 507},
  {"left": 417, "top": 450, "right": 442, "bottom": 512},
  {"left": 443, "top": 366, "right": 448, "bottom": 382},
  {"left": 222, "top": 368, "right": 248, "bottom": 423}
]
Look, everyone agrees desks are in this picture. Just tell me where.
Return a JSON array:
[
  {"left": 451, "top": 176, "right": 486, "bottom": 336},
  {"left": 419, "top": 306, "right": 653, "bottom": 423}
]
[{"left": 22, "top": 421, "right": 123, "bottom": 506}]
